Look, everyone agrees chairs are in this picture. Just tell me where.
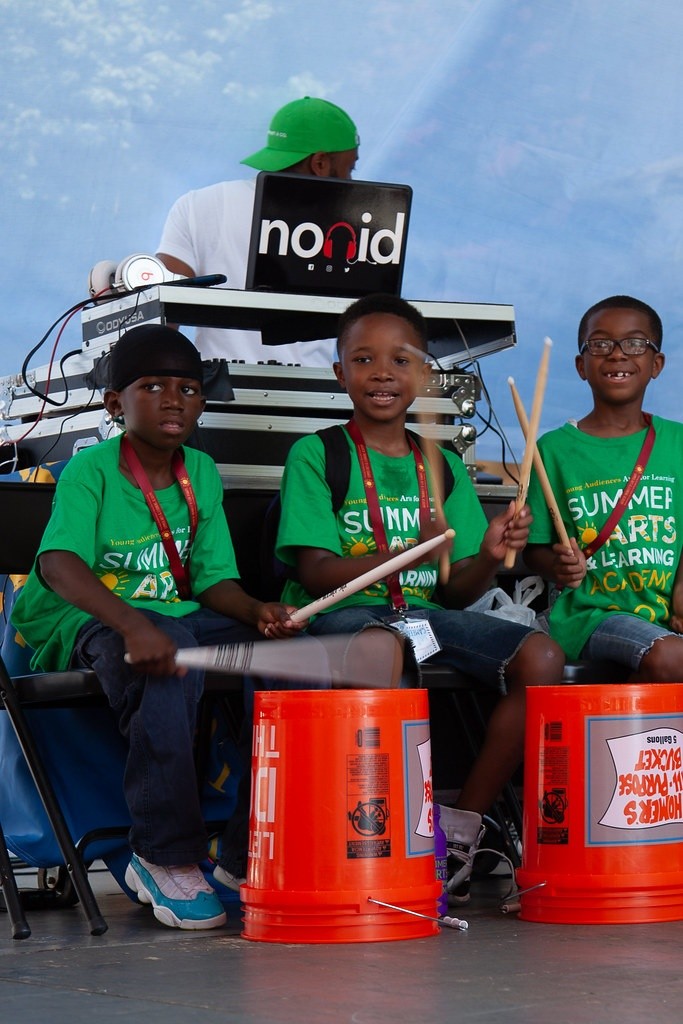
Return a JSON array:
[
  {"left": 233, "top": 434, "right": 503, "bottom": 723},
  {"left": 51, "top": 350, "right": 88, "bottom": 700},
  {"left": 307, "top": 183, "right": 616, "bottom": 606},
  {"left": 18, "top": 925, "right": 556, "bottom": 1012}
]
[{"left": 0, "top": 480, "right": 641, "bottom": 941}]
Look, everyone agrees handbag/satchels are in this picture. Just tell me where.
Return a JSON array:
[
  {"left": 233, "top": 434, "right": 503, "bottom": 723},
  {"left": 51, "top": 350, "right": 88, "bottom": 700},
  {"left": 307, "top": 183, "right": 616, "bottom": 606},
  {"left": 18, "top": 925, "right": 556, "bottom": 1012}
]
[{"left": 462, "top": 575, "right": 546, "bottom": 632}]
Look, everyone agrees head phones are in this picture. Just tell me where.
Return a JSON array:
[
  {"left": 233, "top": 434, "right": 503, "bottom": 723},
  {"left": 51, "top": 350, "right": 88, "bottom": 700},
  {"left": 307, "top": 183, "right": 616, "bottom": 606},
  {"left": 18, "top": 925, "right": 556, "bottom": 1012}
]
[{"left": 90, "top": 254, "right": 169, "bottom": 306}]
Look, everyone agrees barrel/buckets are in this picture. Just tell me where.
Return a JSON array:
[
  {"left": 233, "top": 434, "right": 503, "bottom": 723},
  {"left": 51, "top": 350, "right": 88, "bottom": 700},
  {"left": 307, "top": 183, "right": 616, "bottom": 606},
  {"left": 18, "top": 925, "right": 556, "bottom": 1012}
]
[
  {"left": 490, "top": 682, "right": 683, "bottom": 925},
  {"left": 237, "top": 687, "right": 470, "bottom": 945}
]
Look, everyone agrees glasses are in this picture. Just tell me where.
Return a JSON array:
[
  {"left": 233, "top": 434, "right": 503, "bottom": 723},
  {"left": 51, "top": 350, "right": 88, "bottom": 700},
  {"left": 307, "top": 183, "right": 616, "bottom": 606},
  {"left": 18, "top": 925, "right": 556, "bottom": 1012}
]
[{"left": 579, "top": 336, "right": 660, "bottom": 356}]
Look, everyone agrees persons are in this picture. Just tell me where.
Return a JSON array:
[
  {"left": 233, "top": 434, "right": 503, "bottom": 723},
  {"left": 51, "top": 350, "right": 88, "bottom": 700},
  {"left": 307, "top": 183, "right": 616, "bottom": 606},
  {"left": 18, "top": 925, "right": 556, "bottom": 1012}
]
[
  {"left": 10, "top": 324, "right": 307, "bottom": 930},
  {"left": 522, "top": 294, "right": 683, "bottom": 682},
  {"left": 157, "top": 95, "right": 360, "bottom": 369},
  {"left": 273, "top": 292, "right": 565, "bottom": 902}
]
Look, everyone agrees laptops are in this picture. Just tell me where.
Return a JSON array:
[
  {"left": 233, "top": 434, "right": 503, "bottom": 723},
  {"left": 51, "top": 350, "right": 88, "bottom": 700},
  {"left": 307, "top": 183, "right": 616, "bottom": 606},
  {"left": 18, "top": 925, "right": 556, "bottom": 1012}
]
[{"left": 244, "top": 171, "right": 414, "bottom": 298}]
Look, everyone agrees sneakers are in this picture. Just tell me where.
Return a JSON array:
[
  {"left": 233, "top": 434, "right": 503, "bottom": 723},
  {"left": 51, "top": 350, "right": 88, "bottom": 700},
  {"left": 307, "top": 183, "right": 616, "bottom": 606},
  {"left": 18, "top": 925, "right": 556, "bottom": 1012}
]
[
  {"left": 214, "top": 867, "right": 247, "bottom": 890},
  {"left": 124, "top": 851, "right": 227, "bottom": 931},
  {"left": 441, "top": 822, "right": 484, "bottom": 899}
]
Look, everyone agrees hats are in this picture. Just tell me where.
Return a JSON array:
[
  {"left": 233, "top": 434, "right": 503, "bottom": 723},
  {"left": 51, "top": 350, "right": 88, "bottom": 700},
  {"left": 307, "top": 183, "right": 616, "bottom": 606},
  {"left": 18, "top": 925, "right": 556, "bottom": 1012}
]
[
  {"left": 107, "top": 325, "right": 203, "bottom": 393},
  {"left": 241, "top": 96, "right": 358, "bottom": 171}
]
